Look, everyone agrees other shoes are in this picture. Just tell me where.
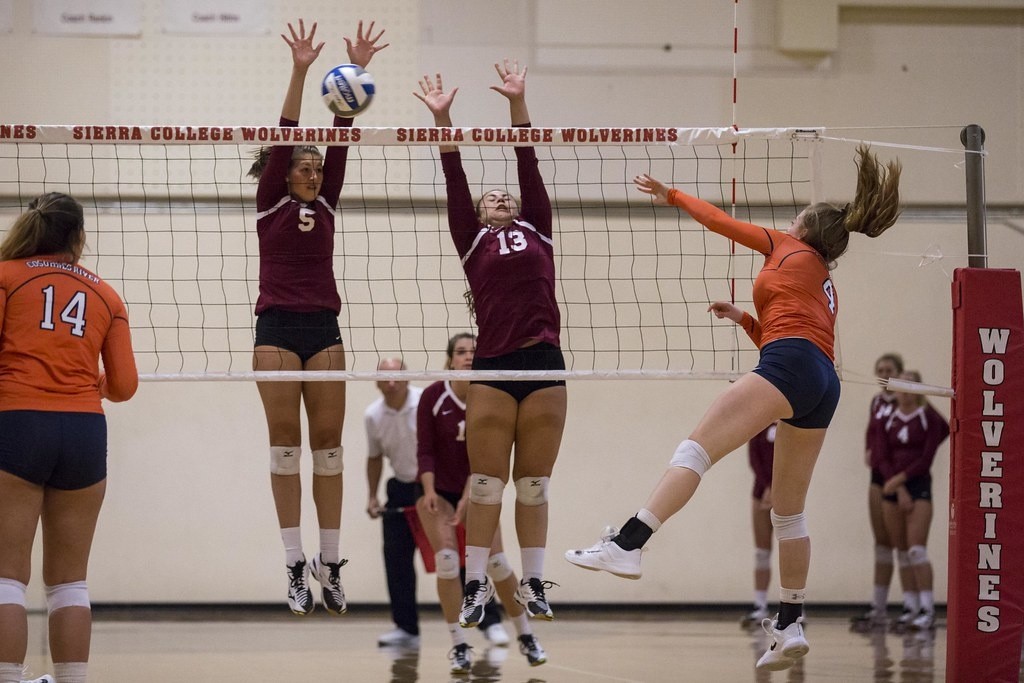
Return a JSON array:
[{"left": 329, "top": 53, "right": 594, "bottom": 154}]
[{"left": 740, "top": 614, "right": 763, "bottom": 629}]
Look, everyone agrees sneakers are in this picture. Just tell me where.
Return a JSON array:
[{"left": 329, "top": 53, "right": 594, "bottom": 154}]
[
  {"left": 448, "top": 643, "right": 478, "bottom": 673},
  {"left": 458, "top": 576, "right": 495, "bottom": 627},
  {"left": 483, "top": 624, "right": 510, "bottom": 647},
  {"left": 285, "top": 553, "right": 315, "bottom": 617},
  {"left": 309, "top": 552, "right": 348, "bottom": 617},
  {"left": 565, "top": 525, "right": 649, "bottom": 580},
  {"left": 755, "top": 613, "right": 808, "bottom": 672},
  {"left": 851, "top": 608, "right": 890, "bottom": 625},
  {"left": 516, "top": 634, "right": 547, "bottom": 667},
  {"left": 513, "top": 577, "right": 560, "bottom": 621},
  {"left": 897, "top": 610, "right": 936, "bottom": 631},
  {"left": 377, "top": 628, "right": 421, "bottom": 650}
]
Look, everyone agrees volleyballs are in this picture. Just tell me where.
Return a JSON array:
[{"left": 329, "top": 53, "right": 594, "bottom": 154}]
[{"left": 321, "top": 63, "right": 375, "bottom": 118}]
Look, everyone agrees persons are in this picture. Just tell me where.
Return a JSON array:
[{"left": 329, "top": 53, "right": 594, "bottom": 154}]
[
  {"left": 0, "top": 191, "right": 138, "bottom": 683},
  {"left": 368, "top": 331, "right": 548, "bottom": 676},
  {"left": 414, "top": 58, "right": 568, "bottom": 627},
  {"left": 741, "top": 354, "right": 949, "bottom": 630},
  {"left": 246, "top": 18, "right": 389, "bottom": 611},
  {"left": 562, "top": 140, "right": 901, "bottom": 673}
]
